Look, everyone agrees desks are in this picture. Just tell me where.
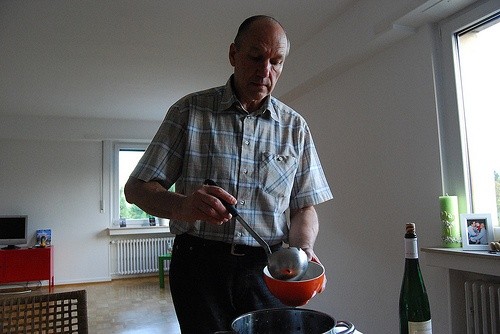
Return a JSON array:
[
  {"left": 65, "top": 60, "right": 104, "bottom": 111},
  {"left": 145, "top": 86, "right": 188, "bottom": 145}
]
[
  {"left": 0, "top": 245, "right": 54, "bottom": 293},
  {"left": 159, "top": 253, "right": 172, "bottom": 289}
]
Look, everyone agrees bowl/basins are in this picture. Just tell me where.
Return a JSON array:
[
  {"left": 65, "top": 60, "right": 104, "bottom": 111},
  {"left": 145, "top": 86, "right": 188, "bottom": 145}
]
[{"left": 264, "top": 262, "right": 325, "bottom": 307}]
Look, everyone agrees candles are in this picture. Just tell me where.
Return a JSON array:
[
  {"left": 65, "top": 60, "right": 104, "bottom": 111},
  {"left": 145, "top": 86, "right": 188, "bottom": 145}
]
[{"left": 439, "top": 193, "right": 462, "bottom": 248}]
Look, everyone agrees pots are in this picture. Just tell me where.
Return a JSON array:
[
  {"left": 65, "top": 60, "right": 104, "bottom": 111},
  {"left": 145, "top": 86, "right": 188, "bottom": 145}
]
[{"left": 215, "top": 308, "right": 355, "bottom": 334}]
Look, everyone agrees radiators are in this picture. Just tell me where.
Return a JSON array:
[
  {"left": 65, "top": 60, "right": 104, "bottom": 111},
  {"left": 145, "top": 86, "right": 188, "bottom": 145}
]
[
  {"left": 111, "top": 236, "right": 175, "bottom": 274},
  {"left": 465, "top": 280, "right": 500, "bottom": 334}
]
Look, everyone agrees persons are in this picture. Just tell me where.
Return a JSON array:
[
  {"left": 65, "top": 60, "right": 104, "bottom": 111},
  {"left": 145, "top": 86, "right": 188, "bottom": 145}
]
[
  {"left": 124, "top": 14, "right": 333, "bottom": 334},
  {"left": 468, "top": 221, "right": 487, "bottom": 244}
]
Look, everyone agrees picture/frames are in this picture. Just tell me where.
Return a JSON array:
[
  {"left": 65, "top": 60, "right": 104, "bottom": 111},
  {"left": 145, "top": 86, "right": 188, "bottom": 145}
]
[{"left": 460, "top": 214, "right": 494, "bottom": 250}]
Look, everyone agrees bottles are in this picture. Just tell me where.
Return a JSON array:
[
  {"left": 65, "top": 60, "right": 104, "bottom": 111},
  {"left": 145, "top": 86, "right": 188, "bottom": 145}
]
[
  {"left": 399, "top": 222, "right": 433, "bottom": 334},
  {"left": 148, "top": 214, "right": 156, "bottom": 226}
]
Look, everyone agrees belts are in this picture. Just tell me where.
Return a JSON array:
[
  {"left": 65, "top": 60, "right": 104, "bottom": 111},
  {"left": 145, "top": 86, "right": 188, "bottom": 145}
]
[{"left": 182, "top": 235, "right": 265, "bottom": 258}]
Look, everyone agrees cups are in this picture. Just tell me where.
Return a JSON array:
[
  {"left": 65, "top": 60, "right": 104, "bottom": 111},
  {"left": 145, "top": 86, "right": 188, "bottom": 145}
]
[{"left": 120, "top": 219, "right": 126, "bottom": 227}]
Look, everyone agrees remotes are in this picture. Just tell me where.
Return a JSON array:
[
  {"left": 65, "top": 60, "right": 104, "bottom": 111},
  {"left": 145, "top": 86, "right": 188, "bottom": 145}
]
[{"left": 28, "top": 245, "right": 32, "bottom": 249}]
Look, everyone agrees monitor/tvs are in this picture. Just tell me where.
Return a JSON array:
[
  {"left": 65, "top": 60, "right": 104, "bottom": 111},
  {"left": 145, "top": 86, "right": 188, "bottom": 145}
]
[{"left": 0, "top": 215, "right": 28, "bottom": 250}]
